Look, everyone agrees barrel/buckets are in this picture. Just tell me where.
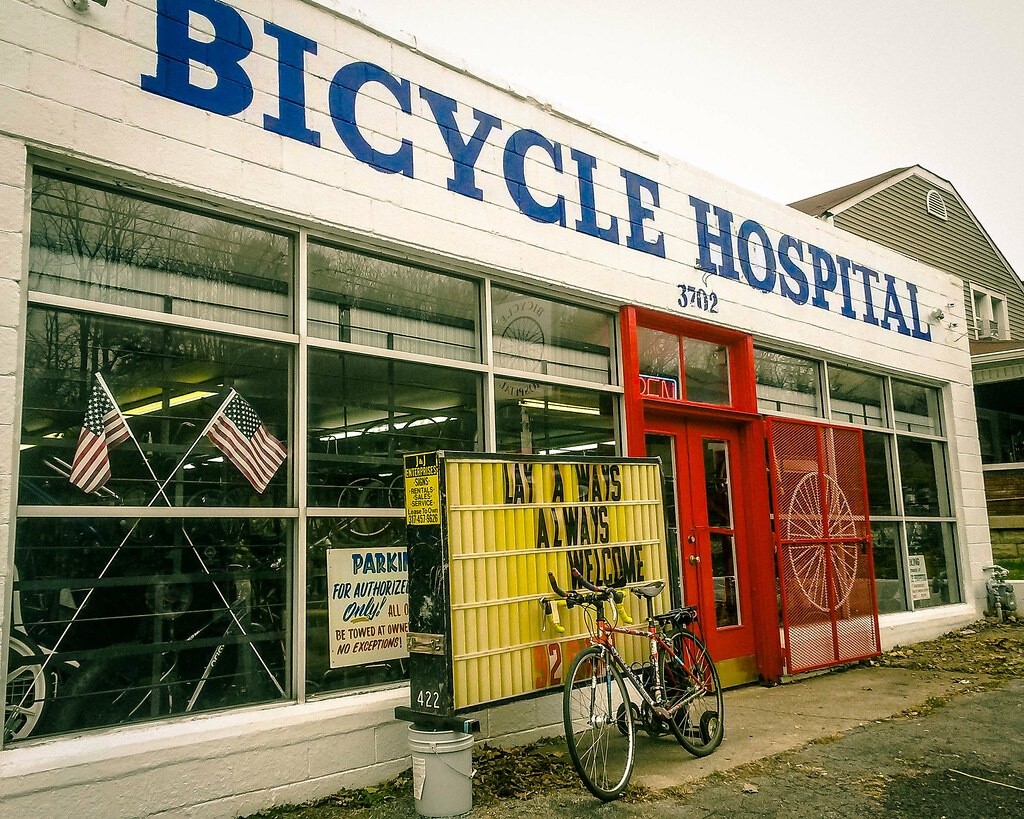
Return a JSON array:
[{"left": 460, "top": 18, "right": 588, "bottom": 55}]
[{"left": 408, "top": 722, "right": 477, "bottom": 817}]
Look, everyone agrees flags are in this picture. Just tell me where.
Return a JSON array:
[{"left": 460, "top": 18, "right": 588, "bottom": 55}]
[
  {"left": 203, "top": 389, "right": 288, "bottom": 494},
  {"left": 69, "top": 373, "right": 131, "bottom": 495}
]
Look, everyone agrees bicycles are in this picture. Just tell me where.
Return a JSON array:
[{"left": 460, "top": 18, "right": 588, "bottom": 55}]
[{"left": 533, "top": 564, "right": 726, "bottom": 801}]
